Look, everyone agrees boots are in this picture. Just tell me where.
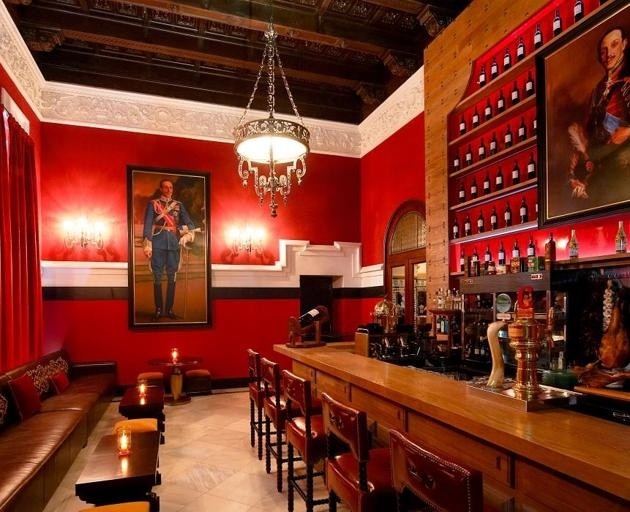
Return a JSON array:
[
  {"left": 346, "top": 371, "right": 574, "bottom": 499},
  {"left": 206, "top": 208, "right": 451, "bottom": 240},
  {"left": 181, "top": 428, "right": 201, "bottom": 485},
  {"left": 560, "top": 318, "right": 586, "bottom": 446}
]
[
  {"left": 154, "top": 285, "right": 163, "bottom": 320},
  {"left": 165, "top": 282, "right": 178, "bottom": 319}
]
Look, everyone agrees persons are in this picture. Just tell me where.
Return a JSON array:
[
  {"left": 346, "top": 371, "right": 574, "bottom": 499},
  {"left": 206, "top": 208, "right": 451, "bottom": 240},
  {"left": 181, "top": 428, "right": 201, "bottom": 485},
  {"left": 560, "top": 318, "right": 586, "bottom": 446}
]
[
  {"left": 561, "top": 27, "right": 630, "bottom": 199},
  {"left": 144, "top": 180, "right": 196, "bottom": 320}
]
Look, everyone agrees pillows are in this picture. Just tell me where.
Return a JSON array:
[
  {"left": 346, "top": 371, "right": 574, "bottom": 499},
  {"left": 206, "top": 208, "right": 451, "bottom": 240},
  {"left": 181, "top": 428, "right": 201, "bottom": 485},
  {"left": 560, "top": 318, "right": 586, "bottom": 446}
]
[
  {"left": 48, "top": 369, "right": 69, "bottom": 395},
  {"left": 8, "top": 373, "right": 41, "bottom": 423}
]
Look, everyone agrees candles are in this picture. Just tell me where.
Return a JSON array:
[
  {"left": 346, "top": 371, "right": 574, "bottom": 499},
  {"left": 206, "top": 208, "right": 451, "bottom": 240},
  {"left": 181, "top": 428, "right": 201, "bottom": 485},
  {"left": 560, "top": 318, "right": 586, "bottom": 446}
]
[
  {"left": 171, "top": 350, "right": 177, "bottom": 358},
  {"left": 119, "top": 430, "right": 128, "bottom": 449},
  {"left": 140, "top": 383, "right": 145, "bottom": 393}
]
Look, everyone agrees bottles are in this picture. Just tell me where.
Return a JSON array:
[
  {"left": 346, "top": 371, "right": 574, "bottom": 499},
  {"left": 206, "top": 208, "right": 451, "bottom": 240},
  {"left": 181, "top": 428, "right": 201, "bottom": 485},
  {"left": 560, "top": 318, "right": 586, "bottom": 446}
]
[
  {"left": 297, "top": 307, "right": 325, "bottom": 327},
  {"left": 553, "top": 9, "right": 562, "bottom": 36},
  {"left": 499, "top": 241, "right": 505, "bottom": 265},
  {"left": 526, "top": 71, "right": 534, "bottom": 97},
  {"left": 491, "top": 205, "right": 497, "bottom": 230},
  {"left": 467, "top": 343, "right": 490, "bottom": 360},
  {"left": 468, "top": 296, "right": 492, "bottom": 312},
  {"left": 616, "top": 221, "right": 627, "bottom": 253},
  {"left": 466, "top": 144, "right": 472, "bottom": 166},
  {"left": 512, "top": 81, "right": 519, "bottom": 105},
  {"left": 534, "top": 115, "right": 537, "bottom": 136},
  {"left": 480, "top": 66, "right": 486, "bottom": 87},
  {"left": 460, "top": 249, "right": 464, "bottom": 272},
  {"left": 527, "top": 240, "right": 535, "bottom": 257},
  {"left": 504, "top": 124, "right": 512, "bottom": 148},
  {"left": 504, "top": 202, "right": 511, "bottom": 226},
  {"left": 536, "top": 202, "right": 539, "bottom": 221},
  {"left": 453, "top": 218, "right": 459, "bottom": 239},
  {"left": 498, "top": 90, "right": 505, "bottom": 112},
  {"left": 471, "top": 179, "right": 478, "bottom": 198},
  {"left": 544, "top": 232, "right": 557, "bottom": 261},
  {"left": 534, "top": 24, "right": 542, "bottom": 49},
  {"left": 465, "top": 215, "right": 470, "bottom": 236},
  {"left": 490, "top": 132, "right": 496, "bottom": 155},
  {"left": 504, "top": 48, "right": 511, "bottom": 70},
  {"left": 574, "top": 0, "right": 584, "bottom": 23},
  {"left": 471, "top": 321, "right": 487, "bottom": 341},
  {"left": 570, "top": 229, "right": 578, "bottom": 258},
  {"left": 429, "top": 289, "right": 461, "bottom": 310},
  {"left": 483, "top": 174, "right": 490, "bottom": 194},
  {"left": 512, "top": 159, "right": 519, "bottom": 185},
  {"left": 512, "top": 240, "right": 520, "bottom": 257},
  {"left": 519, "top": 116, "right": 526, "bottom": 142},
  {"left": 496, "top": 166, "right": 503, "bottom": 190},
  {"left": 485, "top": 99, "right": 492, "bottom": 120},
  {"left": 454, "top": 151, "right": 460, "bottom": 172},
  {"left": 446, "top": 320, "right": 449, "bottom": 333},
  {"left": 520, "top": 197, "right": 528, "bottom": 223},
  {"left": 442, "top": 316, "right": 446, "bottom": 333},
  {"left": 437, "top": 315, "right": 441, "bottom": 333},
  {"left": 460, "top": 117, "right": 466, "bottom": 134},
  {"left": 472, "top": 107, "right": 479, "bottom": 127},
  {"left": 485, "top": 245, "right": 491, "bottom": 261},
  {"left": 458, "top": 182, "right": 465, "bottom": 203},
  {"left": 517, "top": 37, "right": 525, "bottom": 61},
  {"left": 477, "top": 210, "right": 484, "bottom": 233},
  {"left": 472, "top": 247, "right": 478, "bottom": 261},
  {"left": 528, "top": 153, "right": 536, "bottom": 179},
  {"left": 478, "top": 137, "right": 486, "bottom": 161},
  {"left": 550, "top": 352, "right": 564, "bottom": 370},
  {"left": 491, "top": 57, "right": 499, "bottom": 79}
]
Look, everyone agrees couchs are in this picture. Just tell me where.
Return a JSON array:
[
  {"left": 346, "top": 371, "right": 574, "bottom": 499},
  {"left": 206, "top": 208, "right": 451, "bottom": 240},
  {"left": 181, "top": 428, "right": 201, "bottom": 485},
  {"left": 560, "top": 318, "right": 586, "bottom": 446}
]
[
  {"left": 3, "top": 348, "right": 117, "bottom": 436},
  {"left": 1, "top": 374, "right": 88, "bottom": 512}
]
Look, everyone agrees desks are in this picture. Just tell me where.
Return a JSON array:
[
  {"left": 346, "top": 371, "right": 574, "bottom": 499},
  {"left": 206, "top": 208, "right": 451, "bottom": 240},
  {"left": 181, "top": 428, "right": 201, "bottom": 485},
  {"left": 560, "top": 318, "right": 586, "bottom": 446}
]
[
  {"left": 153, "top": 360, "right": 198, "bottom": 406},
  {"left": 74, "top": 429, "right": 161, "bottom": 512},
  {"left": 118, "top": 385, "right": 166, "bottom": 419}
]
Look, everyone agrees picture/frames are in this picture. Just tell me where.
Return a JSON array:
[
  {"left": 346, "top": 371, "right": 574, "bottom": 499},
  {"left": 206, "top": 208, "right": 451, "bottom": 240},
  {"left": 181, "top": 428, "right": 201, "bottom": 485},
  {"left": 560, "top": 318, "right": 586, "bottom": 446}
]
[
  {"left": 126, "top": 165, "right": 212, "bottom": 331},
  {"left": 533, "top": 0, "right": 630, "bottom": 231}
]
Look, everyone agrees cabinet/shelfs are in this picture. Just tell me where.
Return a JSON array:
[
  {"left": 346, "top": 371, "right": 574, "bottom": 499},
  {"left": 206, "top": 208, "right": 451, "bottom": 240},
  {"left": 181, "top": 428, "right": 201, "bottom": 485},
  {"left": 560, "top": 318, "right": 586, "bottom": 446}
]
[
  {"left": 458, "top": 267, "right": 578, "bottom": 375},
  {"left": 446, "top": 0, "right": 630, "bottom": 276}
]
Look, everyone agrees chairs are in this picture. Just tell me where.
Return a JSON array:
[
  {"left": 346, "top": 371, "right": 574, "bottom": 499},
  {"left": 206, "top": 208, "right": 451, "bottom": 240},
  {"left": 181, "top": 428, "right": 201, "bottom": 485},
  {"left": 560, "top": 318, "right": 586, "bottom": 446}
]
[
  {"left": 320, "top": 391, "right": 393, "bottom": 512},
  {"left": 282, "top": 369, "right": 335, "bottom": 512},
  {"left": 387, "top": 427, "right": 516, "bottom": 511},
  {"left": 247, "top": 349, "right": 280, "bottom": 461},
  {"left": 261, "top": 357, "right": 321, "bottom": 493}
]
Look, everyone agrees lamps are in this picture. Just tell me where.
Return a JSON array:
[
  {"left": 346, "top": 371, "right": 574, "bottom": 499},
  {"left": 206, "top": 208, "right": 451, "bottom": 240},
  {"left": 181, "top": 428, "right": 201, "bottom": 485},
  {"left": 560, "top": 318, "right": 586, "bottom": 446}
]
[
  {"left": 116, "top": 426, "right": 132, "bottom": 458},
  {"left": 170, "top": 348, "right": 179, "bottom": 364},
  {"left": 138, "top": 383, "right": 146, "bottom": 397},
  {"left": 231, "top": 15, "right": 310, "bottom": 218}
]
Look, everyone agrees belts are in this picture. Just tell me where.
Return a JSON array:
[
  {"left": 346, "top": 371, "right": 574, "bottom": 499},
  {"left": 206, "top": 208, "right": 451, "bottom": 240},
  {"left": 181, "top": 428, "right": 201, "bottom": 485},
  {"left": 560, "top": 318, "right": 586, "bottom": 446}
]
[{"left": 155, "top": 227, "right": 176, "bottom": 231}]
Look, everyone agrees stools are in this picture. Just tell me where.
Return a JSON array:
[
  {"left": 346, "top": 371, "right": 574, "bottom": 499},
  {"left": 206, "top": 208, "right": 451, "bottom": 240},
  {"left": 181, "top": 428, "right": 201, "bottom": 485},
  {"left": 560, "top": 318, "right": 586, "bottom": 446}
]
[
  {"left": 186, "top": 369, "right": 212, "bottom": 396},
  {"left": 137, "top": 371, "right": 164, "bottom": 397},
  {"left": 74, "top": 501, "right": 155, "bottom": 512},
  {"left": 112, "top": 417, "right": 157, "bottom": 434}
]
